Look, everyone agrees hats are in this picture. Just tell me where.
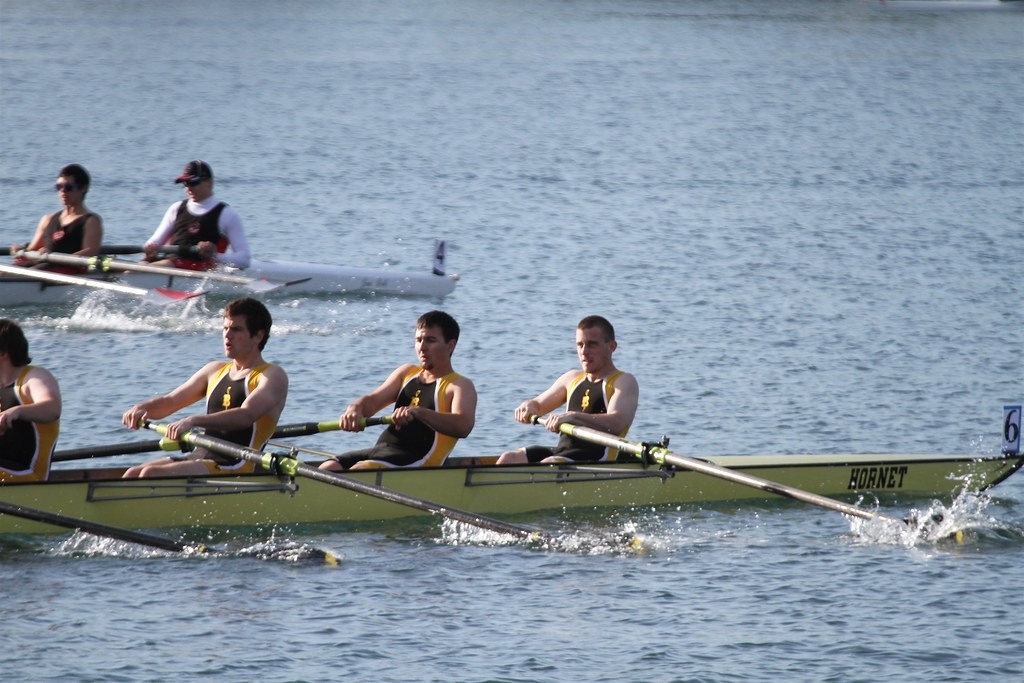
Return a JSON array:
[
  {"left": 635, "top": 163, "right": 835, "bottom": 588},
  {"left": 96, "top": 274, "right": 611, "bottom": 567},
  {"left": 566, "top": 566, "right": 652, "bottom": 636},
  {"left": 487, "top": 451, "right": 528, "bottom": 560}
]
[{"left": 175, "top": 160, "right": 211, "bottom": 181}]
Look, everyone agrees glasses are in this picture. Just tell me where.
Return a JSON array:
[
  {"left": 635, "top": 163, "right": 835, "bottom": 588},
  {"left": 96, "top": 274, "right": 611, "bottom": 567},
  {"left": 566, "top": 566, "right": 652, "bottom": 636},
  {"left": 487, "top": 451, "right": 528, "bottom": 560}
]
[
  {"left": 183, "top": 176, "right": 209, "bottom": 188},
  {"left": 54, "top": 183, "right": 79, "bottom": 192}
]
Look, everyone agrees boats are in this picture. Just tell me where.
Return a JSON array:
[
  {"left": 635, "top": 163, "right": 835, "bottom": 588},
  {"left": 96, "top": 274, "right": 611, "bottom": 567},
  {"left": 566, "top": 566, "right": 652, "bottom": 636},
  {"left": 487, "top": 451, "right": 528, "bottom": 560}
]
[
  {"left": 0, "top": 262, "right": 461, "bottom": 321},
  {"left": 0, "top": 448, "right": 1023, "bottom": 553}
]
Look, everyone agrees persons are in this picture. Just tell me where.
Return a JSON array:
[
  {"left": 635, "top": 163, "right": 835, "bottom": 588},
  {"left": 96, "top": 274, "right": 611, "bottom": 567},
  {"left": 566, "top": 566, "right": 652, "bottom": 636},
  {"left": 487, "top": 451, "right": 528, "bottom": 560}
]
[
  {"left": 123, "top": 160, "right": 250, "bottom": 274},
  {"left": 318, "top": 310, "right": 478, "bottom": 471},
  {"left": 0, "top": 318, "right": 61, "bottom": 484},
  {"left": 496, "top": 315, "right": 638, "bottom": 463},
  {"left": 123, "top": 298, "right": 289, "bottom": 479},
  {"left": 12, "top": 164, "right": 102, "bottom": 274}
]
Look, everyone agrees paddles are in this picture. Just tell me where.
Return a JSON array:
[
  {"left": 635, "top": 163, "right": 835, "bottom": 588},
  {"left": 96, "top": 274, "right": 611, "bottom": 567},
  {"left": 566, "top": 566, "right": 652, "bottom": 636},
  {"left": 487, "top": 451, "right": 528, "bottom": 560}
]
[
  {"left": 0, "top": 242, "right": 215, "bottom": 257},
  {"left": 137, "top": 418, "right": 647, "bottom": 550},
  {"left": 51, "top": 412, "right": 417, "bottom": 464},
  {"left": 0, "top": 262, "right": 212, "bottom": 303},
  {"left": 8, "top": 250, "right": 315, "bottom": 295},
  {"left": 528, "top": 414, "right": 966, "bottom": 546},
  {"left": 0, "top": 501, "right": 343, "bottom": 568}
]
